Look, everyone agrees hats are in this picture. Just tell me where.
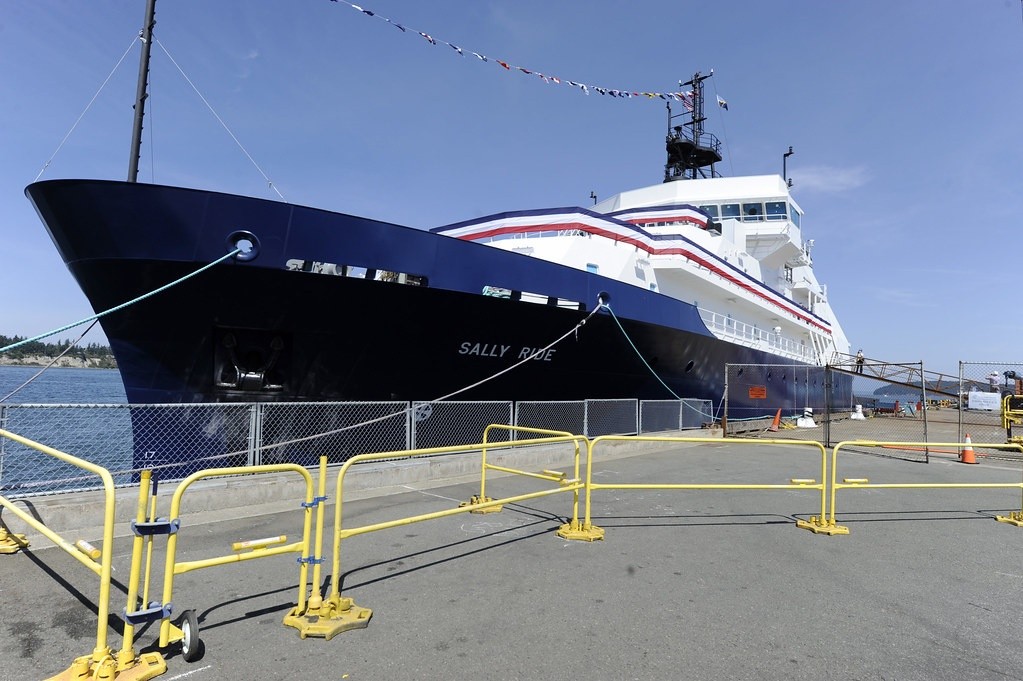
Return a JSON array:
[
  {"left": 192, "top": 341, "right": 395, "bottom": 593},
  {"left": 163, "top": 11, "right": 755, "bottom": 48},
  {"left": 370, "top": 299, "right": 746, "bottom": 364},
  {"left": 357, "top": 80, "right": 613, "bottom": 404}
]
[{"left": 991, "top": 371, "right": 998, "bottom": 375}]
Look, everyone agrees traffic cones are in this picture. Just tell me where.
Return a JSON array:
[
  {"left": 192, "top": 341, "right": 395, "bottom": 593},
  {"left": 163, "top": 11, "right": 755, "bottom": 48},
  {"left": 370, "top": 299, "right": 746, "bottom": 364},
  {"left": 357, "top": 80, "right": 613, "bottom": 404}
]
[
  {"left": 768, "top": 408, "right": 782, "bottom": 431},
  {"left": 958, "top": 433, "right": 979, "bottom": 463}
]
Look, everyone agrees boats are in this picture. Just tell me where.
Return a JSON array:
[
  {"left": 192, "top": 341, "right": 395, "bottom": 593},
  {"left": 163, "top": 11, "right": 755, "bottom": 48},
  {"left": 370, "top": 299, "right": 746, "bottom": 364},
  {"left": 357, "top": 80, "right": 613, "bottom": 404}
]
[{"left": 22, "top": 0, "right": 859, "bottom": 483}]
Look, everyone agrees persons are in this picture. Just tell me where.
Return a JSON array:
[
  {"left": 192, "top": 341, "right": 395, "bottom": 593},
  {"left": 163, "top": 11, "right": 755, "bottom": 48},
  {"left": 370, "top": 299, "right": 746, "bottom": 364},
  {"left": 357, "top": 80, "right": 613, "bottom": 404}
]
[{"left": 855, "top": 349, "right": 864, "bottom": 373}]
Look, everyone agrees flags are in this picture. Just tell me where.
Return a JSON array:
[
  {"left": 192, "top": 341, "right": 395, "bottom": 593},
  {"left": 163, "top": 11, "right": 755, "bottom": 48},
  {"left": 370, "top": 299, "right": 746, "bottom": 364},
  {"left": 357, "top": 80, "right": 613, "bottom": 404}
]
[
  {"left": 642, "top": 90, "right": 694, "bottom": 112},
  {"left": 567, "top": 81, "right": 640, "bottom": 99},
  {"left": 496, "top": 60, "right": 560, "bottom": 84},
  {"left": 717, "top": 95, "right": 727, "bottom": 110},
  {"left": 353, "top": 5, "right": 487, "bottom": 62}
]
